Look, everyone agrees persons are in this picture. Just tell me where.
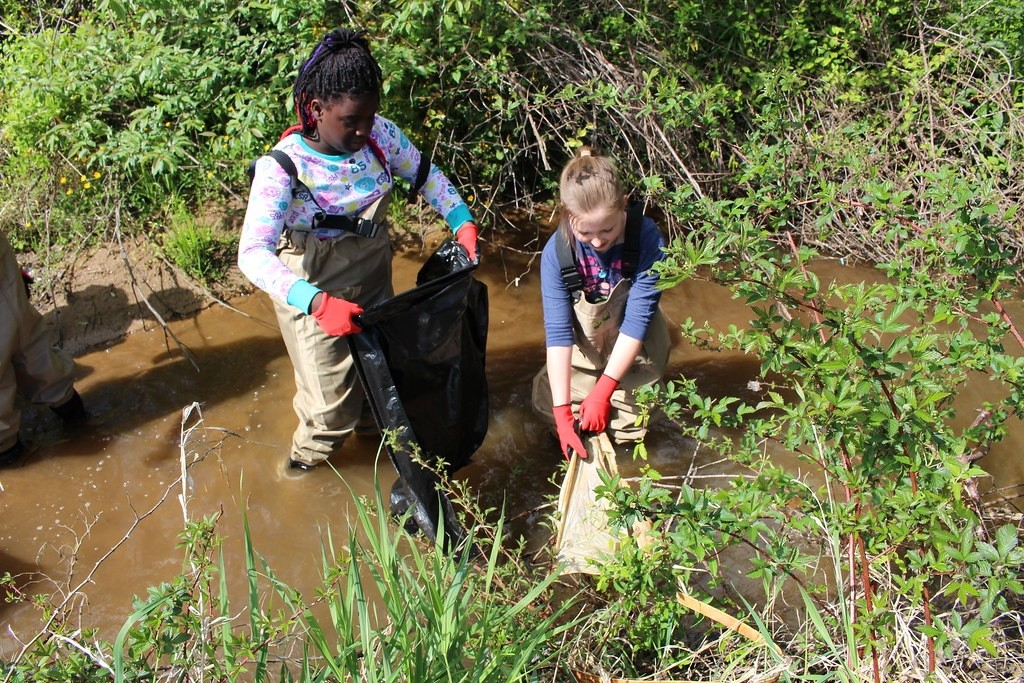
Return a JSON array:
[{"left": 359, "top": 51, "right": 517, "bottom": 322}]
[
  {"left": 0, "top": 285, "right": 288, "bottom": 472},
  {"left": 532, "top": 146, "right": 669, "bottom": 460},
  {"left": 236, "top": 27, "right": 478, "bottom": 471}
]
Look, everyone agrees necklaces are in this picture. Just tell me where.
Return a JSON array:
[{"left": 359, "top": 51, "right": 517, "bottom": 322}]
[{"left": 588, "top": 240, "right": 616, "bottom": 278}]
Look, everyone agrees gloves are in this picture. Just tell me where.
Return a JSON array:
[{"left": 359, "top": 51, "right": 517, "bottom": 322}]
[
  {"left": 456, "top": 223, "right": 477, "bottom": 261},
  {"left": 552, "top": 404, "right": 587, "bottom": 462},
  {"left": 310, "top": 292, "right": 362, "bottom": 336},
  {"left": 579, "top": 374, "right": 620, "bottom": 434}
]
